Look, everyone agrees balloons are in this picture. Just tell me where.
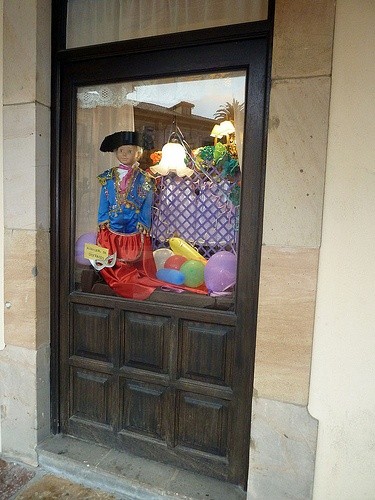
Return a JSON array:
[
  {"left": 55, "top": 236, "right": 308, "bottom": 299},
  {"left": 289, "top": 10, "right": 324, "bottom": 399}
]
[
  {"left": 75, "top": 234, "right": 99, "bottom": 265},
  {"left": 153, "top": 237, "right": 237, "bottom": 293}
]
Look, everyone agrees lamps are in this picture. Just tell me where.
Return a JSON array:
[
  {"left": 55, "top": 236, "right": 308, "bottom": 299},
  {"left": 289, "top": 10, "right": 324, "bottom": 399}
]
[
  {"left": 149, "top": 83, "right": 196, "bottom": 178},
  {"left": 209, "top": 120, "right": 235, "bottom": 138}
]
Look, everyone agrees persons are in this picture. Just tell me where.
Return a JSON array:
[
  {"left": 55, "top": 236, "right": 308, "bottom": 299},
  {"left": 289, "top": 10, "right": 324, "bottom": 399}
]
[{"left": 99, "top": 131, "right": 207, "bottom": 300}]
